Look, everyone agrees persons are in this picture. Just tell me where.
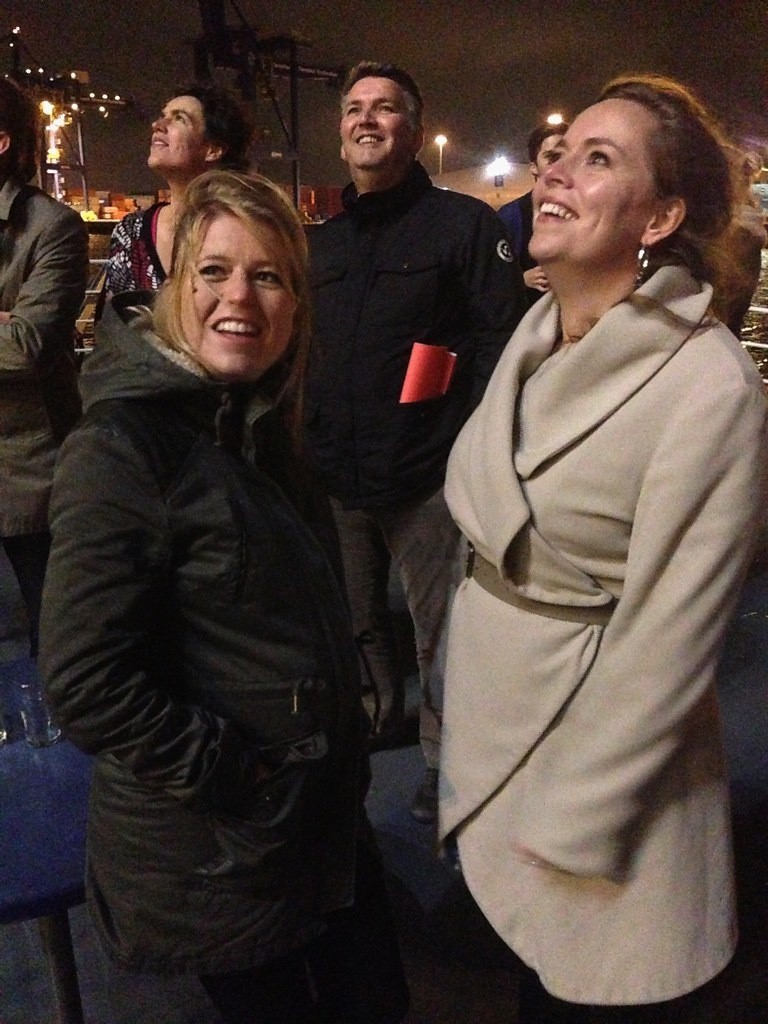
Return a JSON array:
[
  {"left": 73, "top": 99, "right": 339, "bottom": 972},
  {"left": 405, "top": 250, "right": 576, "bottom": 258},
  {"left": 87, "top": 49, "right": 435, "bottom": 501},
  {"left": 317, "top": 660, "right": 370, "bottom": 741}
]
[
  {"left": 497, "top": 123, "right": 568, "bottom": 306},
  {"left": 38, "top": 170, "right": 411, "bottom": 1024},
  {"left": 437, "top": 76, "right": 768, "bottom": 1024},
  {"left": 701, "top": 142, "right": 768, "bottom": 341},
  {"left": 299, "top": 63, "right": 521, "bottom": 825},
  {"left": 0, "top": 74, "right": 91, "bottom": 659},
  {"left": 94, "top": 84, "right": 249, "bottom": 325}
]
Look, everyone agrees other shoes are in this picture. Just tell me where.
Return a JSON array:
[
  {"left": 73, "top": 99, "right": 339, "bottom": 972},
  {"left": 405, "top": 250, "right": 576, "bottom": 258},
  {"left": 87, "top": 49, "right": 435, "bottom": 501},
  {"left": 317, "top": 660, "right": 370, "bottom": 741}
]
[
  {"left": 359, "top": 720, "right": 404, "bottom": 755},
  {"left": 409, "top": 765, "right": 438, "bottom": 823}
]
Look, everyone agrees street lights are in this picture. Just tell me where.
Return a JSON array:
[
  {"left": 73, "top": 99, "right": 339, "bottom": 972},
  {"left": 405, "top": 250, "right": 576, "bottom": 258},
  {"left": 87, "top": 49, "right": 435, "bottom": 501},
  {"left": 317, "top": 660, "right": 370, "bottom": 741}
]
[{"left": 434, "top": 135, "right": 446, "bottom": 186}]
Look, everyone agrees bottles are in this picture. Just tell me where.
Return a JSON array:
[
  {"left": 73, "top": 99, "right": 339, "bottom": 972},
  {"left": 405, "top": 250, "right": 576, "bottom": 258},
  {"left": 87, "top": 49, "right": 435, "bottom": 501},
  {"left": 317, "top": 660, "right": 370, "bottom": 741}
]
[{"left": 14, "top": 655, "right": 62, "bottom": 748}]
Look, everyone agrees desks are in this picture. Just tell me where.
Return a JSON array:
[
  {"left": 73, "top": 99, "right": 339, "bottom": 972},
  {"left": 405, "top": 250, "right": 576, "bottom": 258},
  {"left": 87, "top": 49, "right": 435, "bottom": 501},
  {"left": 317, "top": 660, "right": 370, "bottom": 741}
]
[
  {"left": 0, "top": 736, "right": 95, "bottom": 1024},
  {"left": 0, "top": 656, "right": 49, "bottom": 745}
]
